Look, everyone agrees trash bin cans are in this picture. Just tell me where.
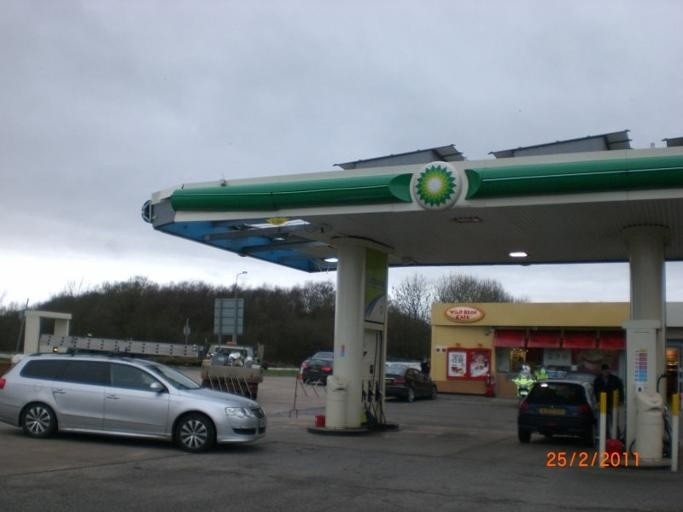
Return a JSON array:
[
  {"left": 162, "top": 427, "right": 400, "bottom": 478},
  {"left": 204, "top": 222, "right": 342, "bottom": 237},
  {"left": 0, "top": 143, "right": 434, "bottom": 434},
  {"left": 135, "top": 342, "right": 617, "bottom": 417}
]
[
  {"left": 327, "top": 376, "right": 349, "bottom": 429},
  {"left": 637, "top": 392, "right": 664, "bottom": 462},
  {"left": 485, "top": 375, "right": 497, "bottom": 397}
]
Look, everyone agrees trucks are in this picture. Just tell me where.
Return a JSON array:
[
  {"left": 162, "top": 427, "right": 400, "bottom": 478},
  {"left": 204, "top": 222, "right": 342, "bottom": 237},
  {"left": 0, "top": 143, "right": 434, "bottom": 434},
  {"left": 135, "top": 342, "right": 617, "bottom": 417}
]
[{"left": 200, "top": 343, "right": 263, "bottom": 400}]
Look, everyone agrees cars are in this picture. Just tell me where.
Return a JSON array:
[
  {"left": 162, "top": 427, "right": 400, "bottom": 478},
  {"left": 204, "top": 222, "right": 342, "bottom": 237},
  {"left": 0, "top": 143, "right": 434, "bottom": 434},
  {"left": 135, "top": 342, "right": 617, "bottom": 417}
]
[
  {"left": 517, "top": 371, "right": 607, "bottom": 445},
  {"left": 301, "top": 351, "right": 334, "bottom": 385},
  {"left": 0, "top": 351, "right": 268, "bottom": 452},
  {"left": 385, "top": 360, "right": 437, "bottom": 403}
]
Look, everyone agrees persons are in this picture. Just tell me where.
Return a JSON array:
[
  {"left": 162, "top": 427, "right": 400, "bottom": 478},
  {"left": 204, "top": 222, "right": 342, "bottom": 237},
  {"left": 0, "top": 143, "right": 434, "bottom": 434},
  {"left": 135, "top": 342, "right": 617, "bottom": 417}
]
[
  {"left": 594, "top": 365, "right": 624, "bottom": 439},
  {"left": 518, "top": 365, "right": 533, "bottom": 379}
]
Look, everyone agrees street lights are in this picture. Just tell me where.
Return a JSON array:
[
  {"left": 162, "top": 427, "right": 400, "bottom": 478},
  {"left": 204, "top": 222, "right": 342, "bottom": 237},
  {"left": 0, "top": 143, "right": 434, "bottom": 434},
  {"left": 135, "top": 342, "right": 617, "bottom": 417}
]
[{"left": 234, "top": 271, "right": 250, "bottom": 343}]
